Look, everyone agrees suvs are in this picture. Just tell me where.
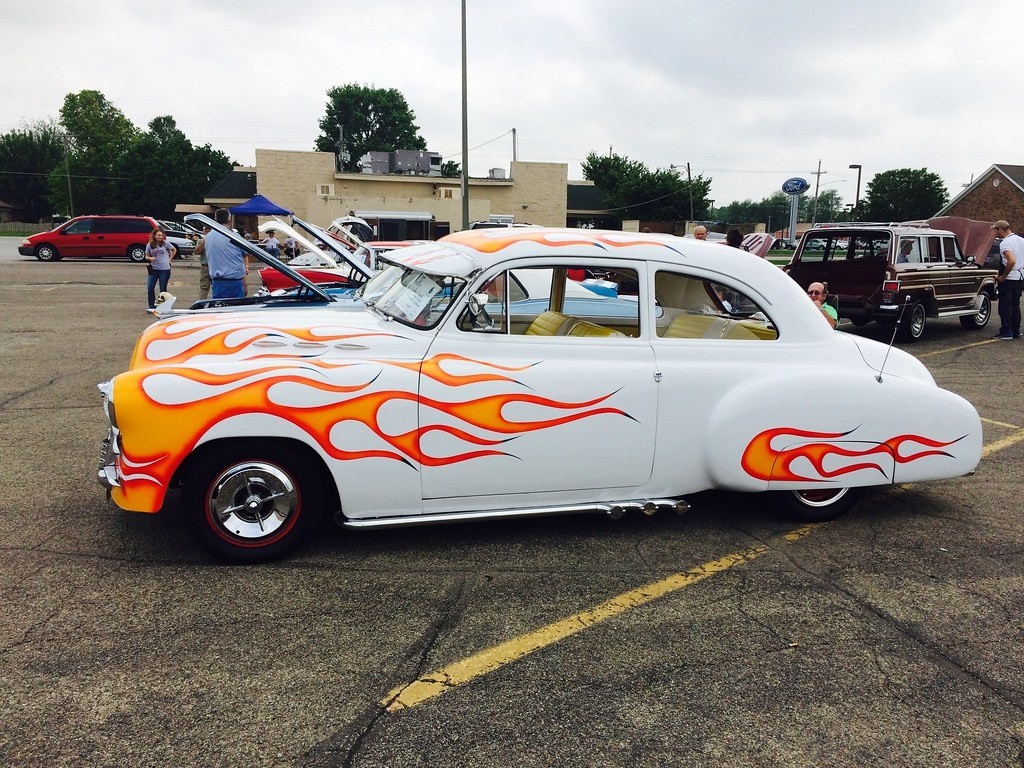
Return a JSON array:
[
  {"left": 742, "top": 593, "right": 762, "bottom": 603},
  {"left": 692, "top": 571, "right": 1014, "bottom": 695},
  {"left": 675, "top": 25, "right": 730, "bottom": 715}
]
[{"left": 781, "top": 221, "right": 999, "bottom": 342}]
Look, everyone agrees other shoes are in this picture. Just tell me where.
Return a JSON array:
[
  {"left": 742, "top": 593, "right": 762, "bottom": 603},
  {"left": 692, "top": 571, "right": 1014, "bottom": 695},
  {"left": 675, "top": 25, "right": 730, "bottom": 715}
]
[
  {"left": 991, "top": 331, "right": 1013, "bottom": 340},
  {"left": 999, "top": 327, "right": 1023, "bottom": 338}
]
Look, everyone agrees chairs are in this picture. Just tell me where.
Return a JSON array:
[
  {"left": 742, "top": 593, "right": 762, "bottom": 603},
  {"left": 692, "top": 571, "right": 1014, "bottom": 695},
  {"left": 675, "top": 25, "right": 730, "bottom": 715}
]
[
  {"left": 565, "top": 319, "right": 629, "bottom": 339},
  {"left": 524, "top": 310, "right": 576, "bottom": 335}
]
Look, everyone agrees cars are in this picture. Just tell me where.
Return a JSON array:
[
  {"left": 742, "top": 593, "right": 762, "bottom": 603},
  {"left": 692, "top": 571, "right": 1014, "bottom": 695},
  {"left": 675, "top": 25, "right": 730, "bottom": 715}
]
[
  {"left": 984, "top": 236, "right": 1009, "bottom": 301},
  {"left": 682, "top": 230, "right": 892, "bottom": 257},
  {"left": 18, "top": 215, "right": 167, "bottom": 263},
  {"left": 96, "top": 225, "right": 984, "bottom": 566},
  {"left": 255, "top": 210, "right": 657, "bottom": 326},
  {"left": 154, "top": 219, "right": 208, "bottom": 260}
]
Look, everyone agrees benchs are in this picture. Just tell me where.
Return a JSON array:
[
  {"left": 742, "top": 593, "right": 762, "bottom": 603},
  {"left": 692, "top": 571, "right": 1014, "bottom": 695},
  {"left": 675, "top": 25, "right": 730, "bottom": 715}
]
[{"left": 660, "top": 314, "right": 775, "bottom": 340}]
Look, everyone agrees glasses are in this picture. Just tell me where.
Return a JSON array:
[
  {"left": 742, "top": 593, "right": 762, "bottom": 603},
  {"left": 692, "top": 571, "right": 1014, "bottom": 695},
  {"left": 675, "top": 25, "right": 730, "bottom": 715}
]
[{"left": 807, "top": 290, "right": 821, "bottom": 296}]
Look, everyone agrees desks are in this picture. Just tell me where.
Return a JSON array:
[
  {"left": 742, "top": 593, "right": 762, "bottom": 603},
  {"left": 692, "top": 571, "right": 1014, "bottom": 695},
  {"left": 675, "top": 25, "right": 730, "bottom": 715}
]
[{"left": 257, "top": 243, "right": 284, "bottom": 251}]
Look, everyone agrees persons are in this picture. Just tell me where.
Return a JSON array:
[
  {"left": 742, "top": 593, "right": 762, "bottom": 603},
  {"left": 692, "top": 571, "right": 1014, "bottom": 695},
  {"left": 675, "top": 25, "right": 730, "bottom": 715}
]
[
  {"left": 282, "top": 236, "right": 301, "bottom": 259},
  {"left": 725, "top": 229, "right": 744, "bottom": 249},
  {"left": 204, "top": 208, "right": 249, "bottom": 298},
  {"left": 990, "top": 219, "right": 1024, "bottom": 339},
  {"left": 898, "top": 240, "right": 915, "bottom": 263},
  {"left": 709, "top": 283, "right": 732, "bottom": 313},
  {"left": 262, "top": 232, "right": 280, "bottom": 259},
  {"left": 194, "top": 226, "right": 212, "bottom": 299},
  {"left": 242, "top": 228, "right": 252, "bottom": 241},
  {"left": 145, "top": 229, "right": 177, "bottom": 312},
  {"left": 807, "top": 282, "right": 839, "bottom": 329},
  {"left": 694, "top": 226, "right": 707, "bottom": 240}
]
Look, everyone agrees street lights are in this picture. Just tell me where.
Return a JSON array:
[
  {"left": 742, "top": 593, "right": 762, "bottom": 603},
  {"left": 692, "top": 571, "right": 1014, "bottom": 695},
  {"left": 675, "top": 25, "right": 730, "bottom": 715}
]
[
  {"left": 670, "top": 161, "right": 694, "bottom": 222},
  {"left": 849, "top": 164, "right": 862, "bottom": 222}
]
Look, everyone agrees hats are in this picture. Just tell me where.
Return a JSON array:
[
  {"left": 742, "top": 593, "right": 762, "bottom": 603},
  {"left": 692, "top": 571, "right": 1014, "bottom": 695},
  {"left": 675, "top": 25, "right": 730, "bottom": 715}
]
[
  {"left": 900, "top": 240, "right": 915, "bottom": 249},
  {"left": 990, "top": 220, "right": 1010, "bottom": 229}
]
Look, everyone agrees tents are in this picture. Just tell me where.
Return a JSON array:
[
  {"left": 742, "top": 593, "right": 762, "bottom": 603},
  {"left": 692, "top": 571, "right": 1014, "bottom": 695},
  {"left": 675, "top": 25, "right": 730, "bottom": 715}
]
[{"left": 228, "top": 194, "right": 296, "bottom": 258}]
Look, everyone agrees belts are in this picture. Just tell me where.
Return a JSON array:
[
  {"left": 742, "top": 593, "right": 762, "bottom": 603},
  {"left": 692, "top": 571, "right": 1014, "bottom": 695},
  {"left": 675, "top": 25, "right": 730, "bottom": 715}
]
[
  {"left": 213, "top": 278, "right": 242, "bottom": 281},
  {"left": 202, "top": 264, "right": 208, "bottom": 267}
]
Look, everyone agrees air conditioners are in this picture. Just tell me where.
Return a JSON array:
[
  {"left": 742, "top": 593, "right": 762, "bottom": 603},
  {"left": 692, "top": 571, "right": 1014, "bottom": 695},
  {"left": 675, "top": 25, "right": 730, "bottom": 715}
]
[
  {"left": 438, "top": 187, "right": 462, "bottom": 198},
  {"left": 315, "top": 182, "right": 335, "bottom": 196}
]
